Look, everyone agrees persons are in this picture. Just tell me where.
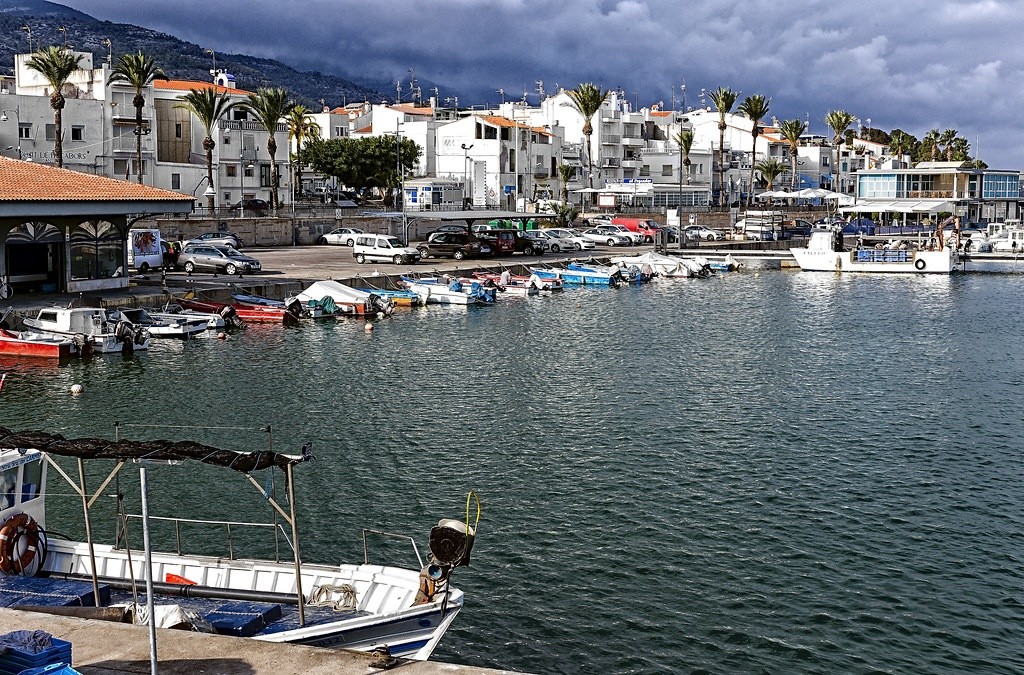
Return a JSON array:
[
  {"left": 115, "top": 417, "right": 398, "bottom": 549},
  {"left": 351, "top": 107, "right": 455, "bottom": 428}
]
[{"left": 500, "top": 269, "right": 512, "bottom": 286}]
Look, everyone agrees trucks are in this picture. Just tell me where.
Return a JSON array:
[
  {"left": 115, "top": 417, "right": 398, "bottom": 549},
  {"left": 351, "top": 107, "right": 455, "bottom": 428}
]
[{"left": 127, "top": 228, "right": 175, "bottom": 274}]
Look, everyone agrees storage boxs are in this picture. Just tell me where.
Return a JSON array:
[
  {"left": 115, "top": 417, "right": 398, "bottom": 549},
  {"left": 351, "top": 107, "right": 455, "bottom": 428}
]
[{"left": 0, "top": 630, "right": 83, "bottom": 675}]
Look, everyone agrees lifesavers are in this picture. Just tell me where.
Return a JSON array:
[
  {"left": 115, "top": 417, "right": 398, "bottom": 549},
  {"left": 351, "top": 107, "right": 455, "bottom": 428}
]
[
  {"left": 915, "top": 259, "right": 925, "bottom": 270},
  {"left": 0, "top": 513, "right": 39, "bottom": 574}
]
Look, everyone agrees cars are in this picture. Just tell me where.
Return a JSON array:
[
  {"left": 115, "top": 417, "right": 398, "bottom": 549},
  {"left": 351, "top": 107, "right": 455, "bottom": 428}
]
[
  {"left": 754, "top": 199, "right": 788, "bottom": 208},
  {"left": 319, "top": 228, "right": 365, "bottom": 247},
  {"left": 417, "top": 234, "right": 492, "bottom": 260},
  {"left": 582, "top": 224, "right": 645, "bottom": 246},
  {"left": 229, "top": 199, "right": 270, "bottom": 211},
  {"left": 426, "top": 225, "right": 491, "bottom": 243},
  {"left": 167, "top": 241, "right": 183, "bottom": 258},
  {"left": 659, "top": 224, "right": 725, "bottom": 242},
  {"left": 814, "top": 216, "right": 875, "bottom": 234},
  {"left": 526, "top": 227, "right": 595, "bottom": 253},
  {"left": 314, "top": 187, "right": 329, "bottom": 192},
  {"left": 582, "top": 214, "right": 612, "bottom": 226}
]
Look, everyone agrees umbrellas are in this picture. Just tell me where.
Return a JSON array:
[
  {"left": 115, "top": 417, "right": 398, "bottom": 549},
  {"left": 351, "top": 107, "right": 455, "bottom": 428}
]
[
  {"left": 786, "top": 191, "right": 803, "bottom": 205},
  {"left": 799, "top": 187, "right": 823, "bottom": 205},
  {"left": 815, "top": 187, "right": 832, "bottom": 205},
  {"left": 773, "top": 190, "right": 788, "bottom": 208},
  {"left": 825, "top": 191, "right": 850, "bottom": 208},
  {"left": 756, "top": 189, "right": 774, "bottom": 207}
]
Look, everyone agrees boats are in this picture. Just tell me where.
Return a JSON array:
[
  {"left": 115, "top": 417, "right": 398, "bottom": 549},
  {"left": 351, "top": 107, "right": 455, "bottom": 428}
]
[
  {"left": 789, "top": 231, "right": 961, "bottom": 272},
  {"left": 529, "top": 261, "right": 655, "bottom": 285},
  {"left": 843, "top": 219, "right": 1024, "bottom": 253},
  {"left": 0, "top": 329, "right": 81, "bottom": 358},
  {"left": 610, "top": 251, "right": 733, "bottom": 279},
  {"left": 106, "top": 300, "right": 227, "bottom": 338},
  {"left": 345, "top": 274, "right": 562, "bottom": 306},
  {"left": 0, "top": 448, "right": 465, "bottom": 661},
  {"left": 175, "top": 290, "right": 353, "bottom": 323},
  {"left": 23, "top": 303, "right": 150, "bottom": 353}
]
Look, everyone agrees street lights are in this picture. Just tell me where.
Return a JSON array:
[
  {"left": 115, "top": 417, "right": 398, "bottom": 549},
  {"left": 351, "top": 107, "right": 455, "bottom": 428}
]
[
  {"left": 225, "top": 120, "right": 244, "bottom": 218},
  {"left": 1, "top": 106, "right": 21, "bottom": 161}
]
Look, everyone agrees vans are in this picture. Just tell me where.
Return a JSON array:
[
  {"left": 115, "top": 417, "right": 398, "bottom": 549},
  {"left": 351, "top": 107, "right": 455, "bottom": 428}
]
[
  {"left": 610, "top": 218, "right": 664, "bottom": 242},
  {"left": 353, "top": 234, "right": 421, "bottom": 265},
  {"left": 735, "top": 218, "right": 814, "bottom": 241},
  {"left": 475, "top": 230, "right": 548, "bottom": 256}
]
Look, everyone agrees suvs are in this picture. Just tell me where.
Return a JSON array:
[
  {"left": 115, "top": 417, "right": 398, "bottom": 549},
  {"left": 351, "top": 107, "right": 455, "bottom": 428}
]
[
  {"left": 182, "top": 232, "right": 245, "bottom": 251},
  {"left": 177, "top": 245, "right": 261, "bottom": 275}
]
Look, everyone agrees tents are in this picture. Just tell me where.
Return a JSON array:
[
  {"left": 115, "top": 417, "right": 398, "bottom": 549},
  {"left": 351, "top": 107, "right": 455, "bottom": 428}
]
[{"left": 840, "top": 201, "right": 958, "bottom": 227}]
